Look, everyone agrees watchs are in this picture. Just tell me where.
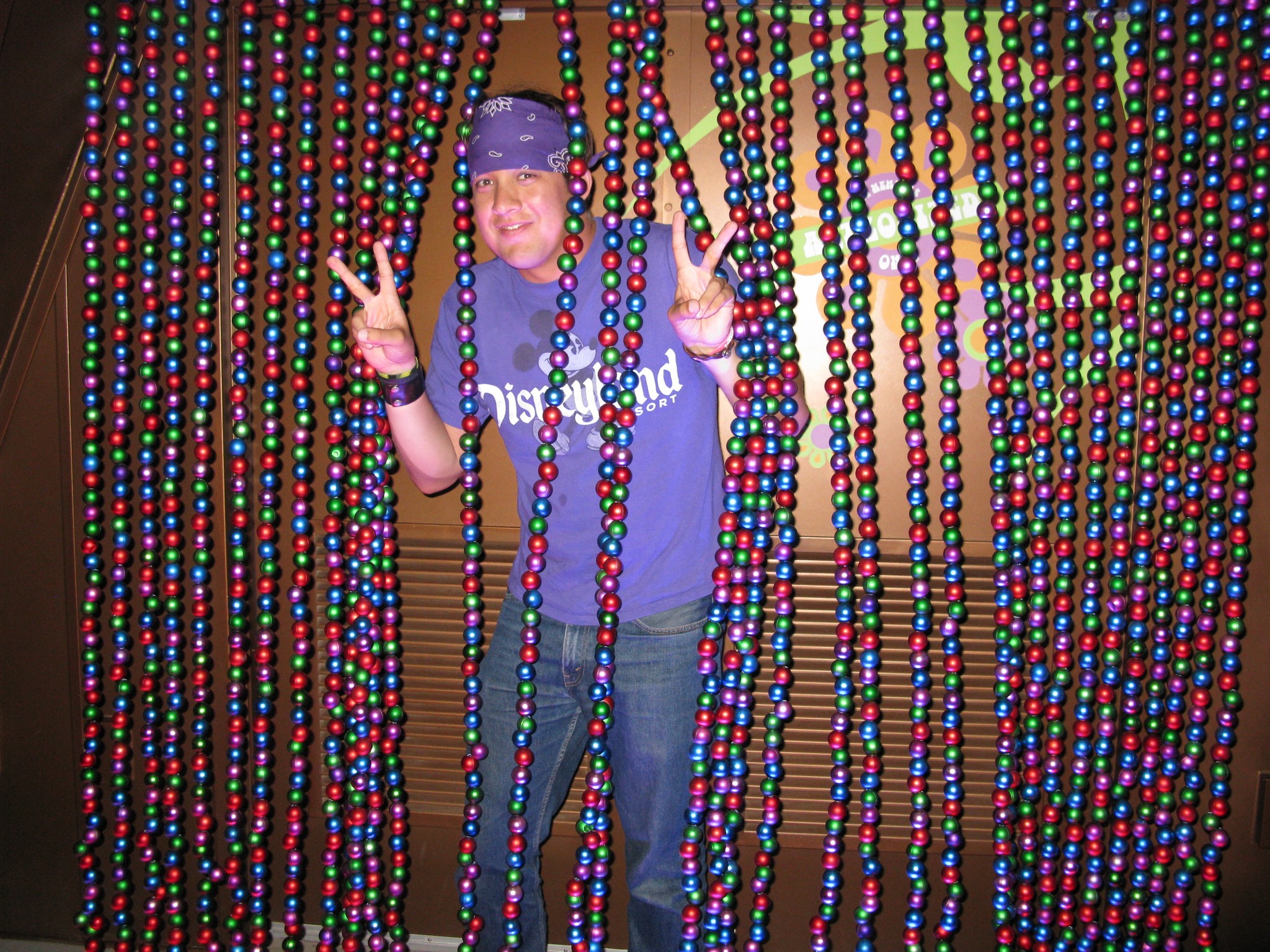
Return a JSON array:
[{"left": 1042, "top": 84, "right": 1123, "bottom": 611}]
[{"left": 682, "top": 325, "right": 735, "bottom": 361}]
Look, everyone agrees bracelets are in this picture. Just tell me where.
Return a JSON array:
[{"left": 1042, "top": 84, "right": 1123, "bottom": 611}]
[{"left": 375, "top": 356, "right": 426, "bottom": 408}]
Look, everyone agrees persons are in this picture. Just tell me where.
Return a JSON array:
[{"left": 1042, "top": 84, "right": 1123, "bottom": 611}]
[{"left": 324, "top": 85, "right": 815, "bottom": 951}]
